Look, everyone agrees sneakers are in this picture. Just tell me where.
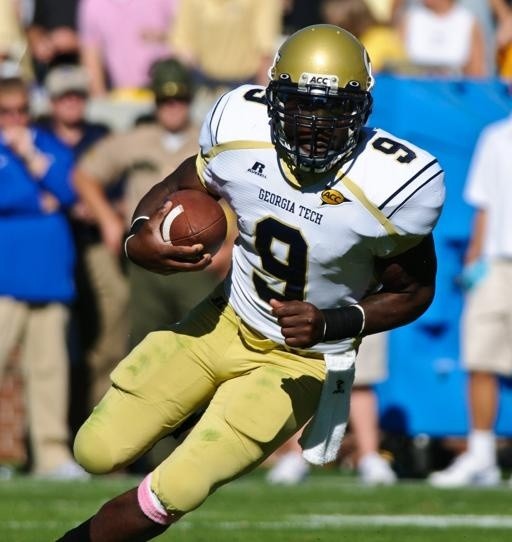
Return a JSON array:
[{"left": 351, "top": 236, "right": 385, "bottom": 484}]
[
  {"left": 267, "top": 452, "right": 308, "bottom": 490},
  {"left": 36, "top": 460, "right": 92, "bottom": 484},
  {"left": 357, "top": 453, "right": 397, "bottom": 488},
  {"left": 428, "top": 450, "right": 502, "bottom": 490}
]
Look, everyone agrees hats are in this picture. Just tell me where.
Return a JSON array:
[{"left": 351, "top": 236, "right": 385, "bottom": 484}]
[
  {"left": 152, "top": 59, "right": 191, "bottom": 99},
  {"left": 46, "top": 64, "right": 90, "bottom": 99}
]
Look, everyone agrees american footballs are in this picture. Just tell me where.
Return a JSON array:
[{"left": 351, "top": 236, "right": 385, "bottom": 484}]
[{"left": 159, "top": 189, "right": 227, "bottom": 261}]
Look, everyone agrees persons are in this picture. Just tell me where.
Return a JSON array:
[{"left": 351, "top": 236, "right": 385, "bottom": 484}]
[
  {"left": 271, "top": 329, "right": 400, "bottom": 488},
  {"left": 1, "top": 0, "right": 280, "bottom": 488},
  {"left": 55, "top": 22, "right": 445, "bottom": 540},
  {"left": 428, "top": 117, "right": 512, "bottom": 488},
  {"left": 323, "top": 0, "right": 512, "bottom": 79}
]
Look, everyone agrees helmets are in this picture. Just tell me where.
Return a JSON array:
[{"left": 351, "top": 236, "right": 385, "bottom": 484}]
[{"left": 266, "top": 24, "right": 373, "bottom": 171}]
